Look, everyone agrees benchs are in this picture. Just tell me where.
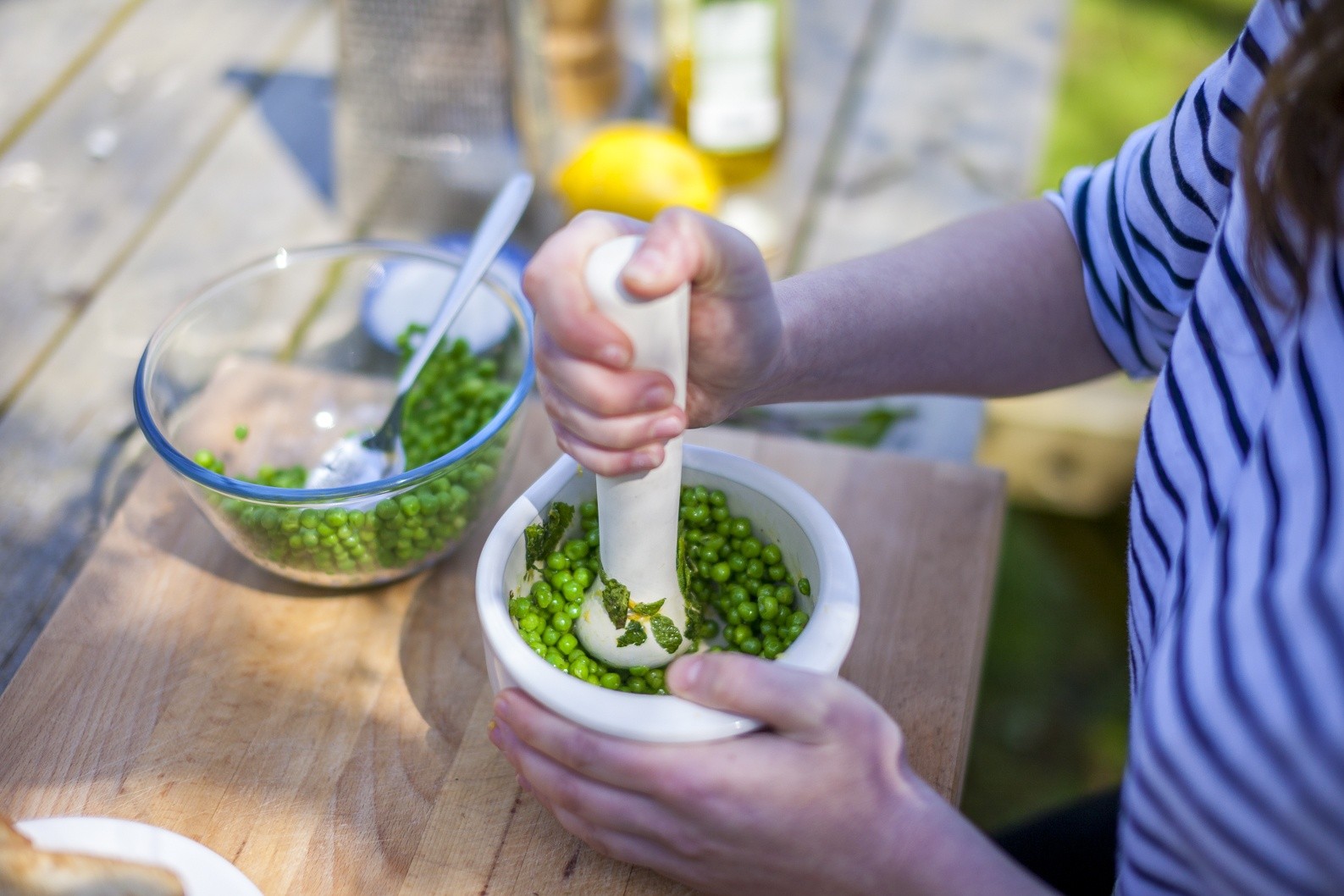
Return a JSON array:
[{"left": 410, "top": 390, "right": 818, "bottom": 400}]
[{"left": 0, "top": 1, "right": 1063, "bottom": 719}]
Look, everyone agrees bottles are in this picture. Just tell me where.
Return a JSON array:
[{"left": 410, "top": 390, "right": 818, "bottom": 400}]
[
  {"left": 530, "top": 0, "right": 621, "bottom": 130},
  {"left": 662, "top": 0, "right": 784, "bottom": 174}
]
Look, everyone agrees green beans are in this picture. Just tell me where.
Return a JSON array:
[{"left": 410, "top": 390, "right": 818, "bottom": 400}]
[
  {"left": 511, "top": 484, "right": 809, "bottom": 696},
  {"left": 193, "top": 325, "right": 517, "bottom": 575}
]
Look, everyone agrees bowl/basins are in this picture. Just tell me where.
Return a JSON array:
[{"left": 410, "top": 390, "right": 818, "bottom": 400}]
[
  {"left": 131, "top": 238, "right": 534, "bottom": 593},
  {"left": 476, "top": 443, "right": 858, "bottom": 748}
]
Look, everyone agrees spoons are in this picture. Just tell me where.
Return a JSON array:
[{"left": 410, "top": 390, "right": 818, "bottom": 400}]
[{"left": 302, "top": 173, "right": 536, "bottom": 510}]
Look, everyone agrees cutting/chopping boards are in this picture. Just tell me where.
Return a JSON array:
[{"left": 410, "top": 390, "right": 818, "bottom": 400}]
[{"left": 0, "top": 360, "right": 1003, "bottom": 896}]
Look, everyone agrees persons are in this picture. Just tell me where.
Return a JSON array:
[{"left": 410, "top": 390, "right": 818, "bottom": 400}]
[{"left": 488, "top": 0, "right": 1344, "bottom": 896}]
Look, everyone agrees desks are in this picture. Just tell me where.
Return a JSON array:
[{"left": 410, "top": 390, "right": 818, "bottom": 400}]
[{"left": 0, "top": 351, "right": 1010, "bottom": 896}]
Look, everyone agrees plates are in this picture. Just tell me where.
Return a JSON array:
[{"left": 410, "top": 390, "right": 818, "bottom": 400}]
[{"left": 362, "top": 233, "right": 539, "bottom": 356}]
